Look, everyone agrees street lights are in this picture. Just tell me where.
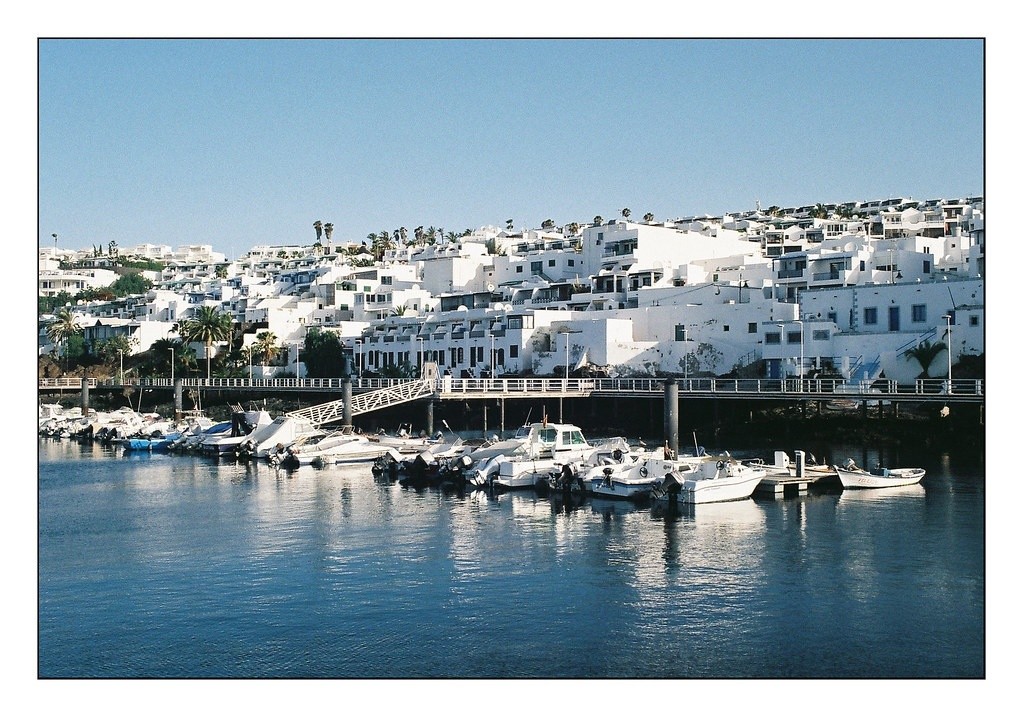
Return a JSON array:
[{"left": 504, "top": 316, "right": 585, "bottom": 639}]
[
  {"left": 680, "top": 329, "right": 688, "bottom": 390},
  {"left": 942, "top": 315, "right": 954, "bottom": 394},
  {"left": 245, "top": 344, "right": 253, "bottom": 379},
  {"left": 117, "top": 349, "right": 123, "bottom": 386},
  {"left": 416, "top": 337, "right": 424, "bottom": 380},
  {"left": 792, "top": 320, "right": 805, "bottom": 393},
  {"left": 355, "top": 340, "right": 363, "bottom": 387},
  {"left": 292, "top": 343, "right": 300, "bottom": 387},
  {"left": 203, "top": 346, "right": 210, "bottom": 386},
  {"left": 167, "top": 348, "right": 174, "bottom": 386},
  {"left": 488, "top": 334, "right": 495, "bottom": 389},
  {"left": 561, "top": 332, "right": 569, "bottom": 387},
  {"left": 776, "top": 324, "right": 785, "bottom": 392}
]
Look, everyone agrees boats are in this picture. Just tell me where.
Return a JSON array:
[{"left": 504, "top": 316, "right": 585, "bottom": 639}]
[{"left": 39, "top": 397, "right": 931, "bottom": 505}]
